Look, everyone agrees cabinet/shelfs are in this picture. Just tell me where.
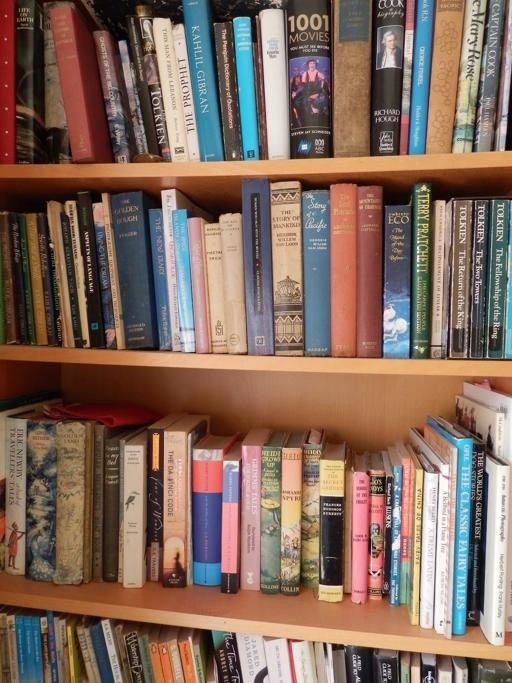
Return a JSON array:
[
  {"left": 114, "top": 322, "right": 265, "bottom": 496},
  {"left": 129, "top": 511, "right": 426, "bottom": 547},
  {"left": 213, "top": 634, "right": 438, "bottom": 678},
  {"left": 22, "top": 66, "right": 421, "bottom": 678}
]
[{"left": 0, "top": 2, "right": 512, "bottom": 663}]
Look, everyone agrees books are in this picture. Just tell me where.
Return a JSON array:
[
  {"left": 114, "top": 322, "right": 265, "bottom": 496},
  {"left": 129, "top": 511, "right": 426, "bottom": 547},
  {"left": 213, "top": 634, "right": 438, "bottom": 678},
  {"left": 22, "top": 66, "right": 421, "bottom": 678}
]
[
  {"left": 0, "top": 178, "right": 512, "bottom": 361},
  {"left": 2, "top": 605, "right": 512, "bottom": 683},
  {"left": 0, "top": 0, "right": 512, "bottom": 163},
  {"left": 0, "top": 379, "right": 511, "bottom": 644}
]
